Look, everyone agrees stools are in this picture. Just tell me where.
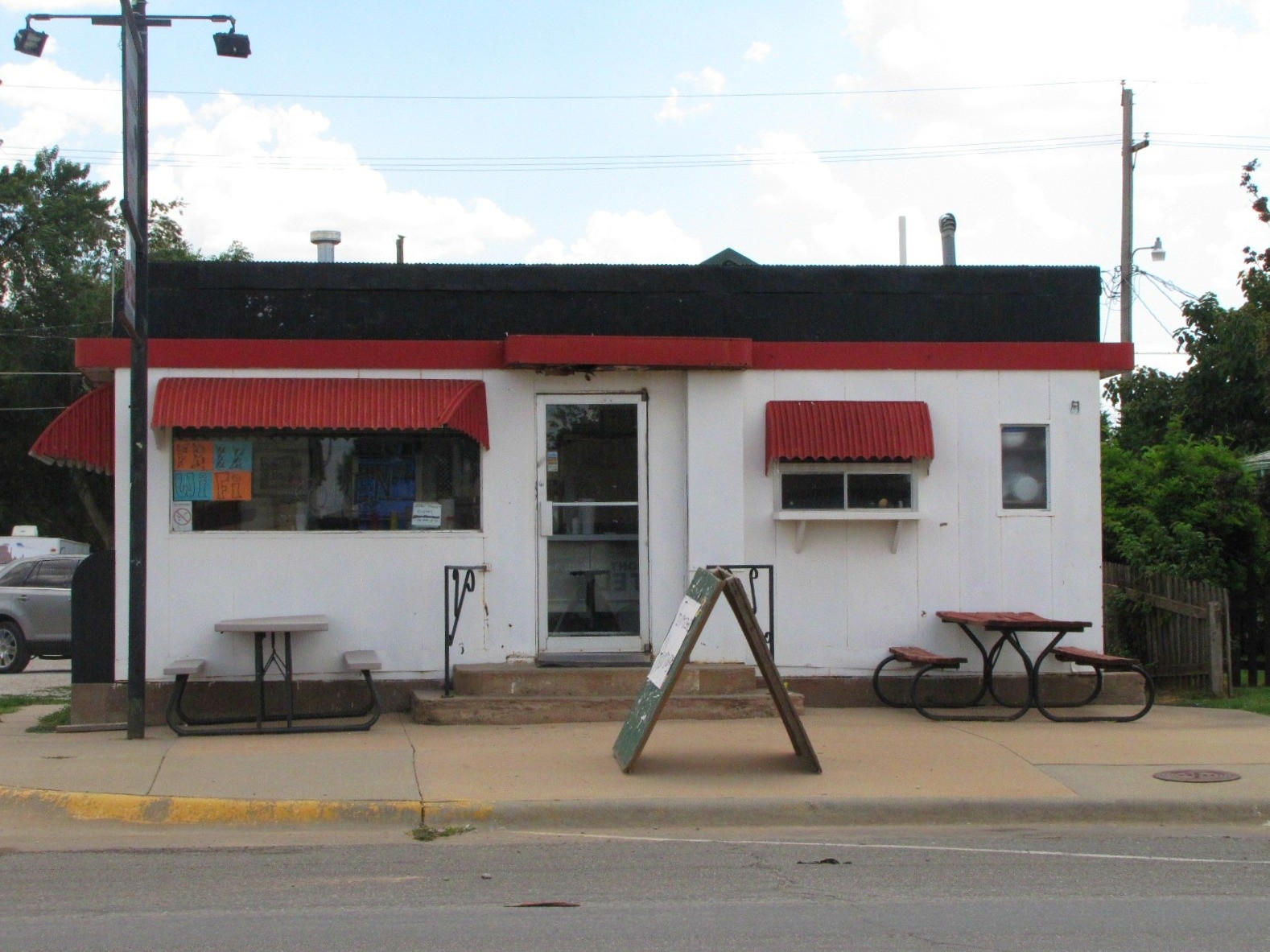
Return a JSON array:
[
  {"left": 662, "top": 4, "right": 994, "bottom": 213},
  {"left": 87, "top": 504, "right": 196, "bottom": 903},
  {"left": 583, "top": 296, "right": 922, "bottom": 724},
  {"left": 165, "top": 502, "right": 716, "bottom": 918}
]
[{"left": 570, "top": 570, "right": 610, "bottom": 632}]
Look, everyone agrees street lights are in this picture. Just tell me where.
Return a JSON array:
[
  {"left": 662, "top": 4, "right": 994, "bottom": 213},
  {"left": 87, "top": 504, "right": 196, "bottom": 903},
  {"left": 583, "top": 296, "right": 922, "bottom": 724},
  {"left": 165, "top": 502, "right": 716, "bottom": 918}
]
[
  {"left": 1119, "top": 238, "right": 1167, "bottom": 414},
  {"left": 12, "top": 0, "right": 253, "bottom": 738}
]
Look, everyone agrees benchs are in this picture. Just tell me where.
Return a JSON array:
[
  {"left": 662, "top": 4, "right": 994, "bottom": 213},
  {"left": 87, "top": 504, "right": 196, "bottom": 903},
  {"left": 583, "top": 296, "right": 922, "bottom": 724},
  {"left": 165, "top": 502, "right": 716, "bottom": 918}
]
[
  {"left": 873, "top": 646, "right": 1155, "bottom": 722},
  {"left": 163, "top": 651, "right": 383, "bottom": 737}
]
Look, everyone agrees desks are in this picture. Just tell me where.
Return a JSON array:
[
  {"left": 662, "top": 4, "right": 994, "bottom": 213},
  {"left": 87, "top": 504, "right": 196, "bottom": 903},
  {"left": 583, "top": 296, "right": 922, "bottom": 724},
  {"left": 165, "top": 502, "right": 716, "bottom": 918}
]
[
  {"left": 214, "top": 616, "right": 328, "bottom": 734},
  {"left": 937, "top": 611, "right": 1091, "bottom": 719}
]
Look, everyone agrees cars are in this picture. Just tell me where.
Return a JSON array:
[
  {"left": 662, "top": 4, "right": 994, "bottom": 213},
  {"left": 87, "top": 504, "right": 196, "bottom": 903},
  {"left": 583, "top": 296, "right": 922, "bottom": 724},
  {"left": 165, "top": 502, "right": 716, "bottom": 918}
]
[{"left": 0, "top": 552, "right": 93, "bottom": 675}]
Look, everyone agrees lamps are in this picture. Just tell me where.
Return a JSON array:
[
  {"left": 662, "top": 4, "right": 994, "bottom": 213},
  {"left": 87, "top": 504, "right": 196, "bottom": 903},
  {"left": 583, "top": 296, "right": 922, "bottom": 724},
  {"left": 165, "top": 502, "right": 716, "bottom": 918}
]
[{"left": 1070, "top": 400, "right": 1081, "bottom": 415}]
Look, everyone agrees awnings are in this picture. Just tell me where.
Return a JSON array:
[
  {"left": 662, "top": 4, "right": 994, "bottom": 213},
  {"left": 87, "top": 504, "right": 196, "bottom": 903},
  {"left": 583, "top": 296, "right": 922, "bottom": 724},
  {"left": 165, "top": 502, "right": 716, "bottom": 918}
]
[
  {"left": 151, "top": 377, "right": 489, "bottom": 451},
  {"left": 29, "top": 382, "right": 113, "bottom": 478},
  {"left": 766, "top": 400, "right": 935, "bottom": 477}
]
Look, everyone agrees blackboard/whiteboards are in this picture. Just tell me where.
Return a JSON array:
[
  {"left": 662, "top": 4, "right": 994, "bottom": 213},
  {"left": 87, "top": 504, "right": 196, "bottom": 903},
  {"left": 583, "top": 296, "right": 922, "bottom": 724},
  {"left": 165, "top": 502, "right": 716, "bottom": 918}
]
[{"left": 613, "top": 566, "right": 822, "bottom": 776}]
